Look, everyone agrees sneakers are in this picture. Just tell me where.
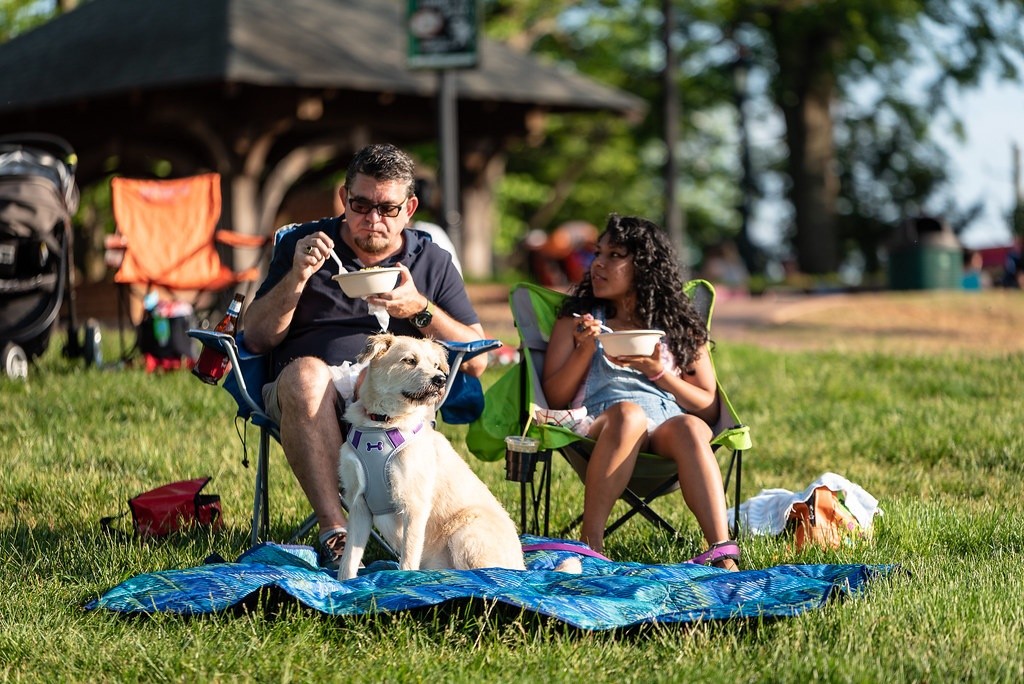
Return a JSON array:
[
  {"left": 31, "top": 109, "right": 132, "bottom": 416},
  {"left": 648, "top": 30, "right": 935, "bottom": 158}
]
[{"left": 314, "top": 530, "right": 367, "bottom": 572}]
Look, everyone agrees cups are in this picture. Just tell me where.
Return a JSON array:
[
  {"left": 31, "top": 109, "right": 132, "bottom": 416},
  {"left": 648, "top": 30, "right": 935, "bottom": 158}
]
[{"left": 503, "top": 436, "right": 540, "bottom": 483}]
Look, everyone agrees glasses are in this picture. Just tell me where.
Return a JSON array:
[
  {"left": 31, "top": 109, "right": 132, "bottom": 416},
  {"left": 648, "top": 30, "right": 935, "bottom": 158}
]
[{"left": 346, "top": 186, "right": 408, "bottom": 217}]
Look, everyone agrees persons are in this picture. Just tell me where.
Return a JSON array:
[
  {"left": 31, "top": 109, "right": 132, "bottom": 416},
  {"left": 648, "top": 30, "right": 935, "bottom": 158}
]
[
  {"left": 243, "top": 143, "right": 488, "bottom": 571},
  {"left": 540, "top": 213, "right": 740, "bottom": 573}
]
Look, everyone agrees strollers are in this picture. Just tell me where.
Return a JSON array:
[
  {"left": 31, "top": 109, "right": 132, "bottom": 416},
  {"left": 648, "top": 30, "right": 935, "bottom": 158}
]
[{"left": 0, "top": 131, "right": 103, "bottom": 385}]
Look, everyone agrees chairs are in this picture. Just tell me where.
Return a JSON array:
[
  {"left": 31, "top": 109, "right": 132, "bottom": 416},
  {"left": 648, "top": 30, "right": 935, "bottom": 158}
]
[
  {"left": 105, "top": 171, "right": 273, "bottom": 367},
  {"left": 185, "top": 217, "right": 503, "bottom": 549},
  {"left": 466, "top": 279, "right": 753, "bottom": 546}
]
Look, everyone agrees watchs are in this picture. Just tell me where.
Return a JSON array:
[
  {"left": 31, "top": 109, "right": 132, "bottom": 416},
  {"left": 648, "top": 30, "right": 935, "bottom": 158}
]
[{"left": 409, "top": 300, "right": 436, "bottom": 329}]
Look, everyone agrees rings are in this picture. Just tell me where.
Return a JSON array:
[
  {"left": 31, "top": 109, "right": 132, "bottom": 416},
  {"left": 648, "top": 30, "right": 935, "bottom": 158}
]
[
  {"left": 577, "top": 322, "right": 586, "bottom": 332},
  {"left": 305, "top": 246, "right": 312, "bottom": 254}
]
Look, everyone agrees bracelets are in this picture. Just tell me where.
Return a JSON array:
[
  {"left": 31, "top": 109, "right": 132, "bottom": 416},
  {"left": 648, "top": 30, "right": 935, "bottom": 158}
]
[{"left": 648, "top": 368, "right": 666, "bottom": 381}]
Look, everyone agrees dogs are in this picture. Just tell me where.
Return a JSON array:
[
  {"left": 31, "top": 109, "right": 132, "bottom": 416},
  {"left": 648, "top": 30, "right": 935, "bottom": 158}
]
[{"left": 338, "top": 332, "right": 582, "bottom": 579}]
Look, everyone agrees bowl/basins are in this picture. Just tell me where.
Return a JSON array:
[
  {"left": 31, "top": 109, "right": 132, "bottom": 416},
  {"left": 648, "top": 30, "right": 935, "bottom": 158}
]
[
  {"left": 596, "top": 330, "right": 666, "bottom": 357},
  {"left": 332, "top": 267, "right": 403, "bottom": 299}
]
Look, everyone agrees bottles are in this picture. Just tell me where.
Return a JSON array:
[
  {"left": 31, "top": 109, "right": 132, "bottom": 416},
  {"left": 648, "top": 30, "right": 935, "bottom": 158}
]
[{"left": 194, "top": 293, "right": 246, "bottom": 382}]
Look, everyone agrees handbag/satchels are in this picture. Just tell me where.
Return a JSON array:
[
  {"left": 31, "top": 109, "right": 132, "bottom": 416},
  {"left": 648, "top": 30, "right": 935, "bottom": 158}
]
[
  {"left": 779, "top": 487, "right": 859, "bottom": 555},
  {"left": 128, "top": 476, "right": 225, "bottom": 541}
]
